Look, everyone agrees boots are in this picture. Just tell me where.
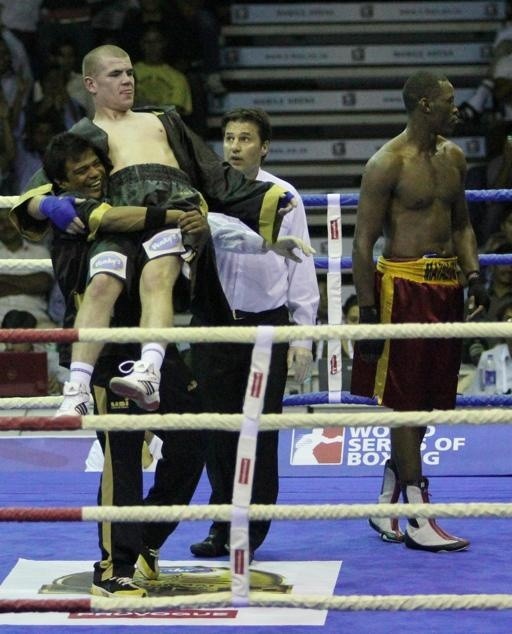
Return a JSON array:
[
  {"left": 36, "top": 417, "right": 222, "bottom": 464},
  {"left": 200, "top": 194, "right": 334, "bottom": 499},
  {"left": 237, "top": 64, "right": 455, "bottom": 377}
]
[
  {"left": 369, "top": 458, "right": 405, "bottom": 544},
  {"left": 396, "top": 476, "right": 470, "bottom": 553}
]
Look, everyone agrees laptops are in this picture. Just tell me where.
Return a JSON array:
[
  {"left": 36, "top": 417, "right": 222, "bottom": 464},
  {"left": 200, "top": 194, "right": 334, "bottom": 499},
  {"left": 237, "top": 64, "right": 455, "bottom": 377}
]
[{"left": 318, "top": 359, "right": 353, "bottom": 392}]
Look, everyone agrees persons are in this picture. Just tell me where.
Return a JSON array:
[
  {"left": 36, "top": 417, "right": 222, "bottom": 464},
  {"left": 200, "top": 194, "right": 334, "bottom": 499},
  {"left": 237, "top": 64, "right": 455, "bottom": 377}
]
[
  {"left": 312, "top": 276, "right": 360, "bottom": 359},
  {"left": 42, "top": 132, "right": 220, "bottom": 601},
  {"left": 1, "top": 1, "right": 235, "bottom": 397},
  {"left": 8, "top": 45, "right": 298, "bottom": 416},
  {"left": 456, "top": 1, "right": 512, "bottom": 397},
  {"left": 352, "top": 70, "right": 491, "bottom": 554},
  {"left": 190, "top": 107, "right": 320, "bottom": 562}
]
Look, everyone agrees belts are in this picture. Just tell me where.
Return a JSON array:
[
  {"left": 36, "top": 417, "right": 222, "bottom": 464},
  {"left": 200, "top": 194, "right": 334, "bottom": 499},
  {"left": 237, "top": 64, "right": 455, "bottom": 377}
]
[{"left": 230, "top": 307, "right": 249, "bottom": 319}]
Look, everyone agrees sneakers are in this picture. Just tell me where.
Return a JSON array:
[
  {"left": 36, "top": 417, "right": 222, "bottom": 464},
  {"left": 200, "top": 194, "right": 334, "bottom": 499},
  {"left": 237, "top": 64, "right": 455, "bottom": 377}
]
[
  {"left": 54, "top": 380, "right": 95, "bottom": 418},
  {"left": 135, "top": 543, "right": 160, "bottom": 580},
  {"left": 109, "top": 361, "right": 161, "bottom": 410},
  {"left": 91, "top": 576, "right": 148, "bottom": 598}
]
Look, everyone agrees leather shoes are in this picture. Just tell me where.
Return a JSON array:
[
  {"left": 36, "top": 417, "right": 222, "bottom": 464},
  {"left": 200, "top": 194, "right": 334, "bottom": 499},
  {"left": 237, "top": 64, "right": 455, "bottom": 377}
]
[{"left": 190, "top": 534, "right": 229, "bottom": 556}]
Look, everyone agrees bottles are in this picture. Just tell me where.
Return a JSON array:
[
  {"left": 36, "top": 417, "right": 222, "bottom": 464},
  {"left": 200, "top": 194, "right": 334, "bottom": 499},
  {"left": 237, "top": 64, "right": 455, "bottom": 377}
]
[{"left": 484, "top": 353, "right": 499, "bottom": 394}]
